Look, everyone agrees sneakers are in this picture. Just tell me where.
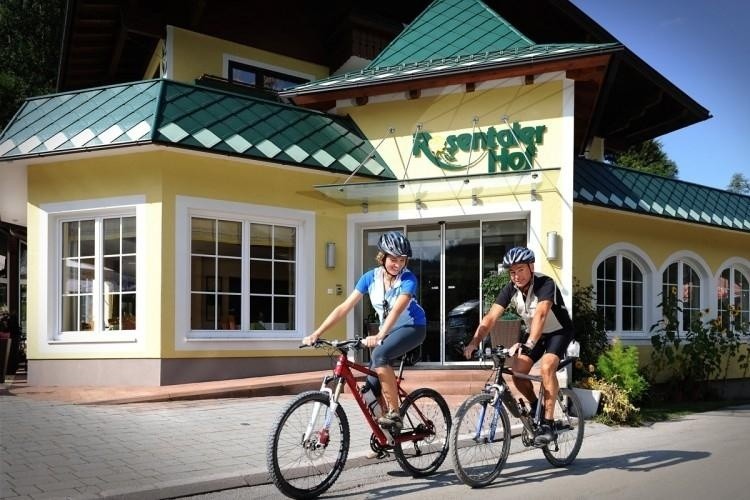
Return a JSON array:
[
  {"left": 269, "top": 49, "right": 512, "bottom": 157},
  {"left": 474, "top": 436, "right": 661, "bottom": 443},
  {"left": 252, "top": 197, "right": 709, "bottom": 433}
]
[
  {"left": 376, "top": 413, "right": 403, "bottom": 428},
  {"left": 365, "top": 451, "right": 379, "bottom": 459},
  {"left": 529, "top": 404, "right": 544, "bottom": 419},
  {"left": 533, "top": 424, "right": 559, "bottom": 445}
]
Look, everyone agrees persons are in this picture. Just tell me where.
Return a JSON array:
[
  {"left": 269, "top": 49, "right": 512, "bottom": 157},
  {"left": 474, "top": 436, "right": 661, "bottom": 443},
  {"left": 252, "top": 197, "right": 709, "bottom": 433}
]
[
  {"left": 463, "top": 246, "right": 574, "bottom": 444},
  {"left": 301, "top": 232, "right": 427, "bottom": 427}
]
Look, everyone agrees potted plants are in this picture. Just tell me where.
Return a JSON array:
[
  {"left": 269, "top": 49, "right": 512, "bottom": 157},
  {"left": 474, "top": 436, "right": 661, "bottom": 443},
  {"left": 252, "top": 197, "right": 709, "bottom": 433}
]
[
  {"left": 672, "top": 341, "right": 693, "bottom": 401},
  {"left": 569, "top": 377, "right": 640, "bottom": 422},
  {"left": 483, "top": 267, "right": 523, "bottom": 355},
  {"left": 691, "top": 328, "right": 711, "bottom": 401}
]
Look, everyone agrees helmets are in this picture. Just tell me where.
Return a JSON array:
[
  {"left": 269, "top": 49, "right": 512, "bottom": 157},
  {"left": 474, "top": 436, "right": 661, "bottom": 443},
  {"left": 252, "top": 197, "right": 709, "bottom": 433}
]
[
  {"left": 502, "top": 246, "right": 535, "bottom": 269},
  {"left": 376, "top": 231, "right": 412, "bottom": 257}
]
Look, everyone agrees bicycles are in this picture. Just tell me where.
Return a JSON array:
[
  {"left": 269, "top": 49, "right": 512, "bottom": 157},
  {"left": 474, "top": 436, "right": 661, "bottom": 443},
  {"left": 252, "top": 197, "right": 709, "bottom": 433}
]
[
  {"left": 449, "top": 343, "right": 586, "bottom": 488},
  {"left": 266, "top": 337, "right": 450, "bottom": 498}
]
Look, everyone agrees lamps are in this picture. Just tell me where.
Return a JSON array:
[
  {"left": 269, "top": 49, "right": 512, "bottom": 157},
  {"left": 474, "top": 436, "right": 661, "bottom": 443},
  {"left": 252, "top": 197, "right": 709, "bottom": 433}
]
[
  {"left": 326, "top": 241, "right": 335, "bottom": 269},
  {"left": 546, "top": 230, "right": 558, "bottom": 261}
]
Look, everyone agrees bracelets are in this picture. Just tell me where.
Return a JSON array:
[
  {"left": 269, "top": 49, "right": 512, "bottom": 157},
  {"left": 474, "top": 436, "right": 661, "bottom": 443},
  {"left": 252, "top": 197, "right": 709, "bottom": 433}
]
[{"left": 526, "top": 340, "right": 536, "bottom": 348}]
